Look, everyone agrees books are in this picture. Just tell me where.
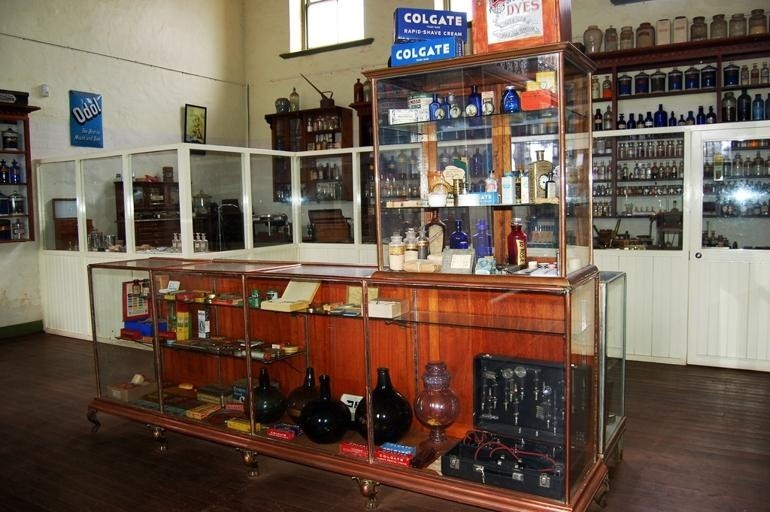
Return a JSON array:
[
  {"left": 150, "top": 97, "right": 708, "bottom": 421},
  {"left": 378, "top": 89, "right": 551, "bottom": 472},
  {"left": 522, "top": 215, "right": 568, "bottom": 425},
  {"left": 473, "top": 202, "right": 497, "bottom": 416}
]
[{"left": 261, "top": 277, "right": 321, "bottom": 313}]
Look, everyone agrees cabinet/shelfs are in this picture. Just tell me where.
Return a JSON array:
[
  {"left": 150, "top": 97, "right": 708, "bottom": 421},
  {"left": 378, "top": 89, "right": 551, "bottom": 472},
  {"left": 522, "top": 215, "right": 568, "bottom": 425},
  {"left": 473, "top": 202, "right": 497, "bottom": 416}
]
[
  {"left": 0, "top": 88, "right": 41, "bottom": 243},
  {"left": 358, "top": 33, "right": 769, "bottom": 220},
  {"left": 361, "top": 40, "right": 600, "bottom": 289},
  {"left": 114, "top": 181, "right": 207, "bottom": 247},
  {"left": 594, "top": 270, "right": 628, "bottom": 479},
  {"left": 85, "top": 256, "right": 609, "bottom": 511},
  {"left": 263, "top": 107, "right": 354, "bottom": 205}
]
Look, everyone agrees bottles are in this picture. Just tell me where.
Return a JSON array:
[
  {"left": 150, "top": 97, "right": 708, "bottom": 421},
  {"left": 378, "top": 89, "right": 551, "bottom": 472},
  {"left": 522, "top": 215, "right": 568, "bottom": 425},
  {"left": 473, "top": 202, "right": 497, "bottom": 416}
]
[
  {"left": 748, "top": 9, "right": 767, "bottom": 35},
  {"left": 646, "top": 141, "right": 654, "bottom": 158},
  {"left": 634, "top": 163, "right": 640, "bottom": 180},
  {"left": 651, "top": 162, "right": 658, "bottom": 180},
  {"left": 1, "top": 160, "right": 9, "bottom": 182},
  {"left": 685, "top": 67, "right": 700, "bottom": 90},
  {"left": 3, "top": 127, "right": 19, "bottom": 152},
  {"left": 706, "top": 107, "right": 717, "bottom": 124},
  {"left": 624, "top": 231, "right": 629, "bottom": 250},
  {"left": 201, "top": 233, "right": 208, "bottom": 252},
  {"left": 651, "top": 70, "right": 666, "bottom": 93},
  {"left": 603, "top": 76, "right": 612, "bottom": 98},
  {"left": 656, "top": 141, "right": 665, "bottom": 157},
  {"left": 425, "top": 211, "right": 447, "bottom": 254},
  {"left": 195, "top": 233, "right": 202, "bottom": 252},
  {"left": 438, "top": 149, "right": 450, "bottom": 170},
  {"left": 752, "top": 94, "right": 764, "bottom": 121},
  {"left": 312, "top": 167, "right": 318, "bottom": 181},
  {"left": 316, "top": 134, "right": 322, "bottom": 150},
  {"left": 332, "top": 164, "right": 339, "bottom": 180},
  {"left": 686, "top": 111, "right": 695, "bottom": 125},
  {"left": 595, "top": 109, "right": 602, "bottom": 131},
  {"left": 724, "top": 159, "right": 732, "bottom": 177},
  {"left": 628, "top": 113, "right": 636, "bottom": 128},
  {"left": 567, "top": 109, "right": 590, "bottom": 134},
  {"left": 404, "top": 231, "right": 418, "bottom": 261},
  {"left": 640, "top": 164, "right": 646, "bottom": 180},
  {"left": 733, "top": 154, "right": 744, "bottom": 177},
  {"left": 722, "top": 92, "right": 737, "bottom": 122},
  {"left": 715, "top": 199, "right": 769, "bottom": 217},
  {"left": 766, "top": 155, "right": 770, "bottom": 178},
  {"left": 620, "top": 26, "right": 634, "bottom": 50},
  {"left": 669, "top": 111, "right": 676, "bottom": 126},
  {"left": 386, "top": 156, "right": 397, "bottom": 180},
  {"left": 609, "top": 201, "right": 613, "bottom": 216},
  {"left": 142, "top": 279, "right": 149, "bottom": 295},
  {"left": 307, "top": 117, "right": 313, "bottom": 133},
  {"left": 397, "top": 151, "right": 408, "bottom": 180},
  {"left": 417, "top": 231, "right": 428, "bottom": 259},
  {"left": 691, "top": 16, "right": 707, "bottom": 41},
  {"left": 761, "top": 63, "right": 769, "bottom": 83},
  {"left": 563, "top": 76, "right": 588, "bottom": 104},
  {"left": 441, "top": 97, "right": 450, "bottom": 119},
  {"left": 312, "top": 115, "right": 340, "bottom": 131},
  {"left": 710, "top": 14, "right": 728, "bottom": 39},
  {"left": 460, "top": 149, "right": 470, "bottom": 176},
  {"left": 729, "top": 14, "right": 747, "bottom": 37},
  {"left": 311, "top": 143, "right": 315, "bottom": 151},
  {"left": 508, "top": 219, "right": 527, "bottom": 268},
  {"left": 704, "top": 180, "right": 770, "bottom": 195},
  {"left": 653, "top": 104, "right": 668, "bottom": 127},
  {"left": 319, "top": 163, "right": 324, "bottom": 180},
  {"left": 703, "top": 231, "right": 709, "bottom": 246},
  {"left": 678, "top": 115, "right": 686, "bottom": 126},
  {"left": 370, "top": 176, "right": 375, "bottom": 198},
  {"left": 666, "top": 140, "right": 675, "bottom": 157},
  {"left": 751, "top": 63, "right": 759, "bottom": 84},
  {"left": 0, "top": 193, "right": 9, "bottom": 216},
  {"left": 765, "top": 93, "right": 770, "bottom": 120},
  {"left": 604, "top": 202, "right": 609, "bottom": 216},
  {"left": 744, "top": 158, "right": 753, "bottom": 177},
  {"left": 593, "top": 162, "right": 598, "bottom": 181},
  {"left": 645, "top": 112, "right": 654, "bottom": 128},
  {"left": 10, "top": 159, "right": 21, "bottom": 183},
  {"left": 429, "top": 93, "right": 439, "bottom": 120},
  {"left": 607, "top": 161, "right": 612, "bottom": 181},
  {"left": 635, "top": 72, "right": 650, "bottom": 95},
  {"left": 604, "top": 28, "right": 618, "bottom": 52},
  {"left": 485, "top": 171, "right": 498, "bottom": 192},
  {"left": 387, "top": 178, "right": 420, "bottom": 199},
  {"left": 670, "top": 200, "right": 678, "bottom": 212},
  {"left": 617, "top": 184, "right": 683, "bottom": 196},
  {"left": 599, "top": 161, "right": 605, "bottom": 180},
  {"left": 697, "top": 105, "right": 706, "bottom": 124},
  {"left": 618, "top": 142, "right": 626, "bottom": 160},
  {"left": 668, "top": 67, "right": 683, "bottom": 91},
  {"left": 325, "top": 163, "right": 332, "bottom": 179},
  {"left": 528, "top": 151, "right": 552, "bottom": 200},
  {"left": 592, "top": 76, "right": 600, "bottom": 99},
  {"left": 568, "top": 156, "right": 579, "bottom": 199},
  {"left": 592, "top": 187, "right": 597, "bottom": 197},
  {"left": 753, "top": 151, "right": 764, "bottom": 177},
  {"left": 363, "top": 77, "right": 373, "bottom": 102},
  {"left": 380, "top": 174, "right": 387, "bottom": 198},
  {"left": 636, "top": 114, "right": 644, "bottom": 128},
  {"left": 618, "top": 114, "right": 626, "bottom": 129},
  {"left": 545, "top": 172, "right": 556, "bottom": 197},
  {"left": 516, "top": 170, "right": 526, "bottom": 203},
  {"left": 679, "top": 161, "right": 684, "bottom": 180},
  {"left": 484, "top": 147, "right": 492, "bottom": 177},
  {"left": 354, "top": 79, "right": 364, "bottom": 103},
  {"left": 408, "top": 150, "right": 419, "bottom": 179},
  {"left": 9, "top": 191, "right": 25, "bottom": 215},
  {"left": 172, "top": 233, "right": 179, "bottom": 249},
  {"left": 598, "top": 201, "right": 603, "bottom": 217},
  {"left": 676, "top": 140, "right": 684, "bottom": 158},
  {"left": 671, "top": 162, "right": 677, "bottom": 180},
  {"left": 646, "top": 164, "right": 651, "bottom": 180},
  {"left": 623, "top": 163, "right": 628, "bottom": 180},
  {"left": 500, "top": 85, "right": 521, "bottom": 114},
  {"left": 636, "top": 23, "right": 655, "bottom": 48},
  {"left": 12, "top": 218, "right": 27, "bottom": 240},
  {"left": 592, "top": 202, "right": 598, "bottom": 217},
  {"left": 604, "top": 106, "right": 612, "bottom": 130},
  {"left": 446, "top": 192, "right": 455, "bottom": 207},
  {"left": 636, "top": 142, "right": 644, "bottom": 157},
  {"left": 451, "top": 149, "right": 459, "bottom": 164},
  {"left": 627, "top": 142, "right": 635, "bottom": 158},
  {"left": 468, "top": 85, "right": 482, "bottom": 115},
  {"left": 596, "top": 184, "right": 613, "bottom": 196},
  {"left": 322, "top": 133, "right": 328, "bottom": 150},
  {"left": 471, "top": 147, "right": 482, "bottom": 177},
  {"left": 704, "top": 161, "right": 711, "bottom": 177},
  {"left": 388, "top": 235, "right": 405, "bottom": 270},
  {"left": 177, "top": 235, "right": 182, "bottom": 253},
  {"left": 701, "top": 65, "right": 717, "bottom": 89},
  {"left": 289, "top": 88, "right": 299, "bottom": 112},
  {"left": 618, "top": 75, "right": 632, "bottom": 96},
  {"left": 473, "top": 219, "right": 492, "bottom": 259},
  {"left": 741, "top": 65, "right": 749, "bottom": 85},
  {"left": 737, "top": 89, "right": 751, "bottom": 121},
  {"left": 533, "top": 225, "right": 556, "bottom": 248},
  {"left": 665, "top": 162, "right": 671, "bottom": 180},
  {"left": 335, "top": 183, "right": 342, "bottom": 200},
  {"left": 724, "top": 62, "right": 740, "bottom": 87},
  {"left": 710, "top": 231, "right": 716, "bottom": 247},
  {"left": 450, "top": 220, "right": 469, "bottom": 249},
  {"left": 132, "top": 279, "right": 141, "bottom": 295},
  {"left": 658, "top": 163, "right": 665, "bottom": 180}
]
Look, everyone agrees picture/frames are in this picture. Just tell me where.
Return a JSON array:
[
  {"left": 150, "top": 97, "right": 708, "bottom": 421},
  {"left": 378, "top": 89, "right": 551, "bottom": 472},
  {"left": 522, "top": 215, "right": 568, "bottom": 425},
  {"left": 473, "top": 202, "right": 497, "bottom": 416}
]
[{"left": 185, "top": 104, "right": 208, "bottom": 156}]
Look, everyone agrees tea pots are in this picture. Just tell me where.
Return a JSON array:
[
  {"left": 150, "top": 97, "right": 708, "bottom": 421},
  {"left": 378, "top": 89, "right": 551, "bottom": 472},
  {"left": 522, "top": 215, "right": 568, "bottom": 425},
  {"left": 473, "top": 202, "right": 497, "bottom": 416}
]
[{"left": 298, "top": 72, "right": 335, "bottom": 107}]
